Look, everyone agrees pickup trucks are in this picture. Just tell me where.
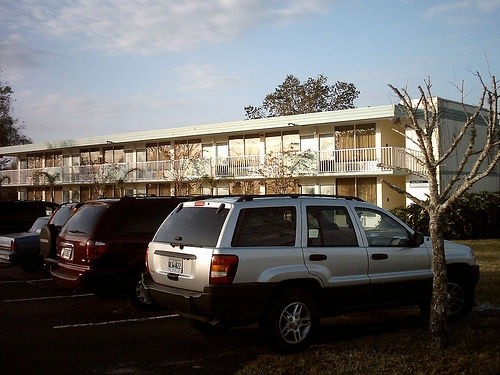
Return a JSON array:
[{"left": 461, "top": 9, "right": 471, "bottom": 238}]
[{"left": 0, "top": 215, "right": 50, "bottom": 268}]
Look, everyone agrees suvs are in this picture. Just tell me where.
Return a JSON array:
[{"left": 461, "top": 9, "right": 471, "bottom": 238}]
[
  {"left": 35, "top": 200, "right": 82, "bottom": 269},
  {"left": 50, "top": 195, "right": 202, "bottom": 310},
  {"left": 146, "top": 193, "right": 480, "bottom": 354}
]
